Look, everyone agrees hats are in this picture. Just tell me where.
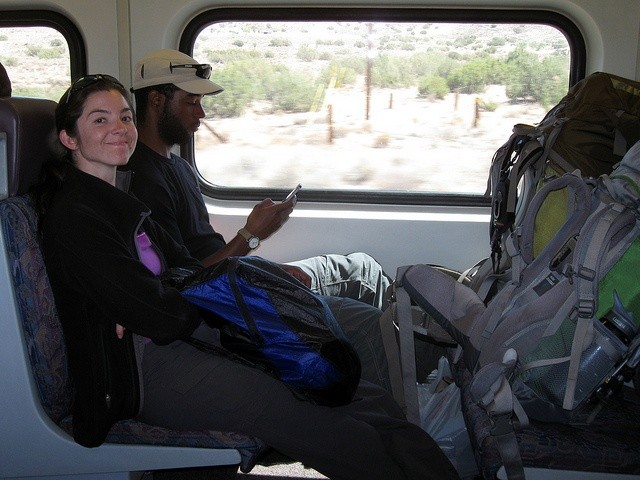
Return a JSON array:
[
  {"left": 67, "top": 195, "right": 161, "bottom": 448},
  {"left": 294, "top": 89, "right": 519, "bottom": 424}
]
[{"left": 132, "top": 48, "right": 224, "bottom": 95}]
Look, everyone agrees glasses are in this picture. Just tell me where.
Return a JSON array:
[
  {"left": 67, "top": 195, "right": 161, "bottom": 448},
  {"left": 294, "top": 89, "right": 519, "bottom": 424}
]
[
  {"left": 171, "top": 64, "right": 212, "bottom": 79},
  {"left": 65, "top": 74, "right": 124, "bottom": 104}
]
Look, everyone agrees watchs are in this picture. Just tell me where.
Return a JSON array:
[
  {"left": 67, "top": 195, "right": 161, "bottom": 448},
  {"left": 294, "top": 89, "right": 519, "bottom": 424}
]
[{"left": 247, "top": 235, "right": 261, "bottom": 250}]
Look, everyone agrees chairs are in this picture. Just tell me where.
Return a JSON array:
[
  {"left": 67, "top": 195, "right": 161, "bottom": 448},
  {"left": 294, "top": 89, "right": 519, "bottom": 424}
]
[
  {"left": 444, "top": 346, "right": 472, "bottom": 385},
  {"left": 459, "top": 379, "right": 640, "bottom": 478},
  {"left": 0, "top": 99, "right": 266, "bottom": 480}
]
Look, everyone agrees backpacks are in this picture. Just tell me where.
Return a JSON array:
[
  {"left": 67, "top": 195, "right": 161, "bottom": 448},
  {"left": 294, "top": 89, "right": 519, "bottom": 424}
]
[
  {"left": 457, "top": 72, "right": 640, "bottom": 307},
  {"left": 379, "top": 142, "right": 639, "bottom": 479}
]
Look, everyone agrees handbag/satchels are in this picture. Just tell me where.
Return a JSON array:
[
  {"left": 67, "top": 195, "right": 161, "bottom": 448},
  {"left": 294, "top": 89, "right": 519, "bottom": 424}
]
[{"left": 165, "top": 255, "right": 362, "bottom": 407}]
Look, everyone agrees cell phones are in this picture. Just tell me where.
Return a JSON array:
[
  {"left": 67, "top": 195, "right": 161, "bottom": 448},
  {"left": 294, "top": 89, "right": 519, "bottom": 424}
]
[{"left": 283, "top": 185, "right": 300, "bottom": 202}]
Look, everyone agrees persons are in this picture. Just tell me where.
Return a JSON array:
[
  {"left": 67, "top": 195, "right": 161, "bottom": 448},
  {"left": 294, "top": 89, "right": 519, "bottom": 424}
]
[
  {"left": 117, "top": 50, "right": 456, "bottom": 391},
  {"left": 36, "top": 74, "right": 458, "bottom": 479}
]
